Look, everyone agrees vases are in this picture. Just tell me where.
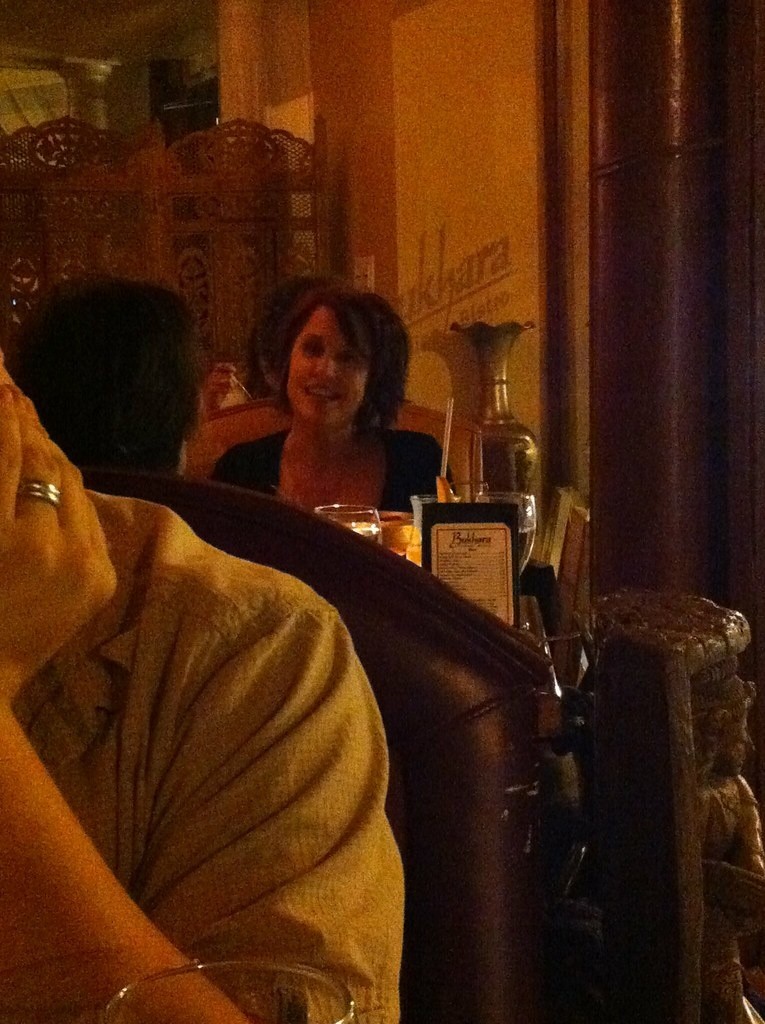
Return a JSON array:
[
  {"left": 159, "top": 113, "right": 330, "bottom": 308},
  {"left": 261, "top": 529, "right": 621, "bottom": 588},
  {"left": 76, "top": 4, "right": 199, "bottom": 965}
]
[{"left": 429, "top": 311, "right": 551, "bottom": 575}]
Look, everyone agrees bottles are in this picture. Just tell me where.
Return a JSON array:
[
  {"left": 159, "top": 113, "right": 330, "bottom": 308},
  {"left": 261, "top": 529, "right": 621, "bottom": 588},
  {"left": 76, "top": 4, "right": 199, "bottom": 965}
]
[{"left": 450, "top": 319, "right": 540, "bottom": 493}]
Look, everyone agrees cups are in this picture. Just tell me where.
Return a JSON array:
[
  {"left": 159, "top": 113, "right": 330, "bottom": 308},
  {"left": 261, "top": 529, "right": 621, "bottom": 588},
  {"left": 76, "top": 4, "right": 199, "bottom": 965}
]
[
  {"left": 406, "top": 493, "right": 461, "bottom": 573},
  {"left": 474, "top": 491, "right": 536, "bottom": 572},
  {"left": 377, "top": 511, "right": 414, "bottom": 556},
  {"left": 313, "top": 503, "right": 383, "bottom": 548},
  {"left": 104, "top": 958, "right": 355, "bottom": 1024}
]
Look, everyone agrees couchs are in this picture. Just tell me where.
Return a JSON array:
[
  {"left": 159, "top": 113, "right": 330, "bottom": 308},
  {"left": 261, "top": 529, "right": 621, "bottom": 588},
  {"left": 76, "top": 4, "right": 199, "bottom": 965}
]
[{"left": 84, "top": 466, "right": 554, "bottom": 1024}]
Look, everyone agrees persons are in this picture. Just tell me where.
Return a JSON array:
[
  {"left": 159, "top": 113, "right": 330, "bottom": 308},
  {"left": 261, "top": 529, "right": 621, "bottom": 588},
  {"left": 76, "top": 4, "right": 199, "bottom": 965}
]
[
  {"left": 206, "top": 282, "right": 457, "bottom": 516},
  {"left": 3, "top": 276, "right": 209, "bottom": 478},
  {"left": 0, "top": 353, "right": 407, "bottom": 1024}
]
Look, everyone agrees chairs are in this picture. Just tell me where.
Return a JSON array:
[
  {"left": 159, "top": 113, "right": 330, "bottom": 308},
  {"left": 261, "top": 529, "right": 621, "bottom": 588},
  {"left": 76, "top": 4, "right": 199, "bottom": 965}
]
[{"left": 180, "top": 386, "right": 483, "bottom": 512}]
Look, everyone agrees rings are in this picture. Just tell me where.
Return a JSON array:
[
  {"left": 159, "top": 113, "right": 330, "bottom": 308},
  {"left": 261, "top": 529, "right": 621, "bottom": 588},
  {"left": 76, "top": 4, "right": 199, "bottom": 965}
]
[{"left": 17, "top": 479, "right": 61, "bottom": 507}]
